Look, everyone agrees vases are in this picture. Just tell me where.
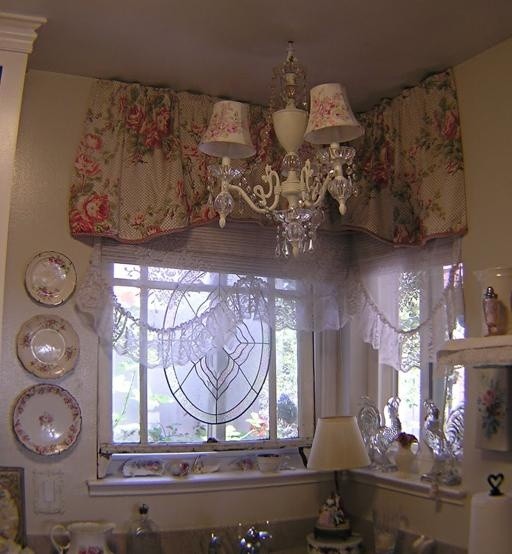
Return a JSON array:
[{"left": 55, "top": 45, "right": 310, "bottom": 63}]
[{"left": 394, "top": 442, "right": 414, "bottom": 479}]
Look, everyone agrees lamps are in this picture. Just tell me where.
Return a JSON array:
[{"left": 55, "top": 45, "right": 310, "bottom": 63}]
[
  {"left": 306, "top": 415, "right": 373, "bottom": 510},
  {"left": 469, "top": 363, "right": 508, "bottom": 453},
  {"left": 197, "top": 39, "right": 365, "bottom": 258}
]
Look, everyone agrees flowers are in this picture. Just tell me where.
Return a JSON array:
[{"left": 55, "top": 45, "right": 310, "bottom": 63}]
[{"left": 392, "top": 432, "right": 419, "bottom": 446}]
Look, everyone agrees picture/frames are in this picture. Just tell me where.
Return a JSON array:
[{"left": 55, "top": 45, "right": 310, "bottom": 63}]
[{"left": 0, "top": 465, "right": 27, "bottom": 550}]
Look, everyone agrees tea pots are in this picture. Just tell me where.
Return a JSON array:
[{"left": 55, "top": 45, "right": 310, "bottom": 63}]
[{"left": 51, "top": 522, "right": 116, "bottom": 554}]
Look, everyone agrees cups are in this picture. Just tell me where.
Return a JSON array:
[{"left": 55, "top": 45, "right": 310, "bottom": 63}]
[
  {"left": 233, "top": 521, "right": 273, "bottom": 554},
  {"left": 372, "top": 509, "right": 401, "bottom": 554}
]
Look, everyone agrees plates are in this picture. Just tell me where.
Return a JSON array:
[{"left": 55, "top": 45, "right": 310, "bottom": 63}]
[
  {"left": 24, "top": 250, "right": 76, "bottom": 306},
  {"left": 16, "top": 313, "right": 81, "bottom": 379},
  {"left": 12, "top": 382, "right": 82, "bottom": 456}
]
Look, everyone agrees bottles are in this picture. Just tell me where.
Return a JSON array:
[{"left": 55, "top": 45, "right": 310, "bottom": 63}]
[
  {"left": 482, "top": 287, "right": 499, "bottom": 336},
  {"left": 207, "top": 528, "right": 220, "bottom": 554},
  {"left": 127, "top": 504, "right": 161, "bottom": 554}
]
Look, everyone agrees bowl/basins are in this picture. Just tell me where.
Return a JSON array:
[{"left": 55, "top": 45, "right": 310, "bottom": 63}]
[{"left": 166, "top": 462, "right": 190, "bottom": 477}]
